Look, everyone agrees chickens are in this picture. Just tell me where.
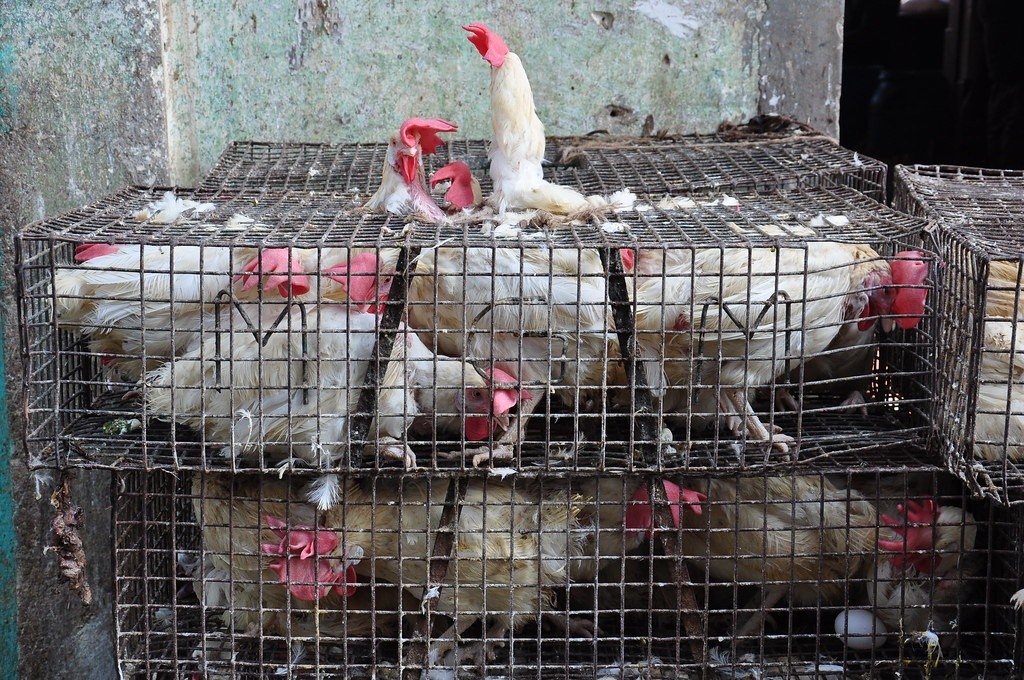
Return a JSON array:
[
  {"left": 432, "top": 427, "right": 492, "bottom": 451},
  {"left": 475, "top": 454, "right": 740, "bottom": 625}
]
[{"left": 44, "top": 22, "right": 1024, "bottom": 669}]
